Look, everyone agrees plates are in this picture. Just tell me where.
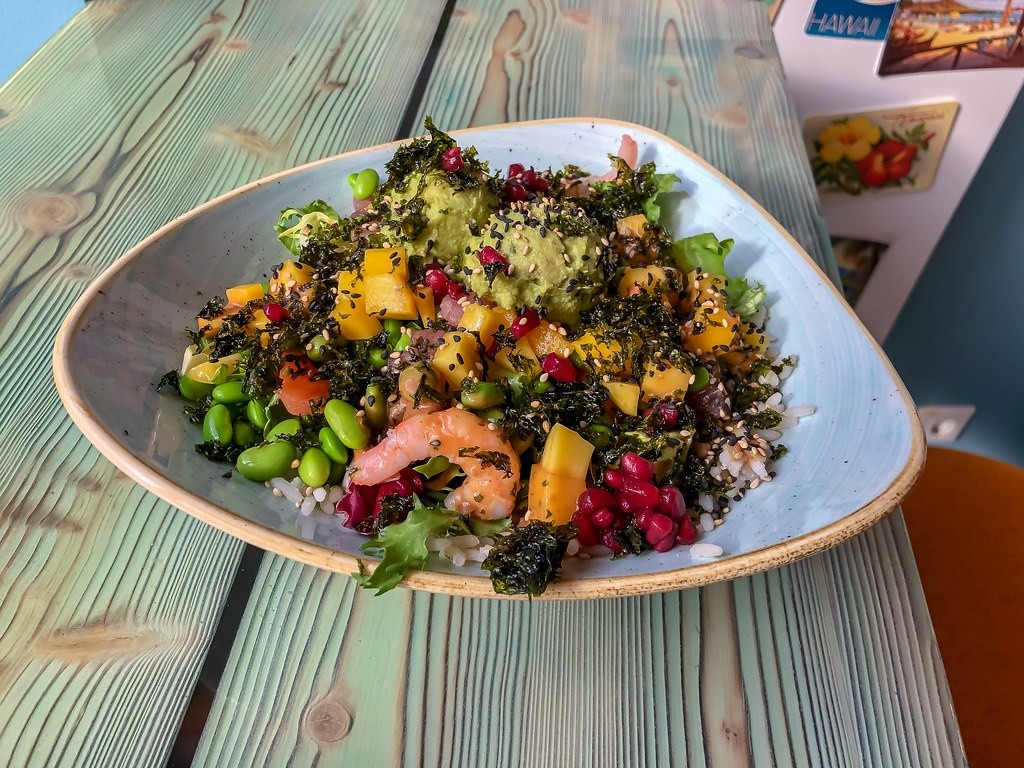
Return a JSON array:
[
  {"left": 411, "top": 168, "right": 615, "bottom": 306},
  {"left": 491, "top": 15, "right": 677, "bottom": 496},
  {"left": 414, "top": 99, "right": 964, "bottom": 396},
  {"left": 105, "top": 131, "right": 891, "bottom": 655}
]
[{"left": 51, "top": 116, "right": 928, "bottom": 602}]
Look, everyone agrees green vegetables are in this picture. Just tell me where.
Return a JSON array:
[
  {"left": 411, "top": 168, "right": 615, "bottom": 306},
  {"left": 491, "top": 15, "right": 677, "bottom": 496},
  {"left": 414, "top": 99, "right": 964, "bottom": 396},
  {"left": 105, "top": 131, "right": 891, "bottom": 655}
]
[{"left": 273, "top": 115, "right": 768, "bottom": 598}]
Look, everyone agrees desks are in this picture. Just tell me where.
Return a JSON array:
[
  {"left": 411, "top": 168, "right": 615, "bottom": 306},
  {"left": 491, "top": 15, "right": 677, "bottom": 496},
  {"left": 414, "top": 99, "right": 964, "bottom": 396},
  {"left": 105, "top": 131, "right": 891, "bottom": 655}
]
[{"left": 0, "top": 0, "right": 973, "bottom": 767}]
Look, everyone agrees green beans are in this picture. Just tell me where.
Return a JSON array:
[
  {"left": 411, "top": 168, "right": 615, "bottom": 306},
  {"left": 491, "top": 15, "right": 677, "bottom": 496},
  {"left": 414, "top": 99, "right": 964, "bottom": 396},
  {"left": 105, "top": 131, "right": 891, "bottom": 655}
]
[{"left": 179, "top": 318, "right": 615, "bottom": 488}]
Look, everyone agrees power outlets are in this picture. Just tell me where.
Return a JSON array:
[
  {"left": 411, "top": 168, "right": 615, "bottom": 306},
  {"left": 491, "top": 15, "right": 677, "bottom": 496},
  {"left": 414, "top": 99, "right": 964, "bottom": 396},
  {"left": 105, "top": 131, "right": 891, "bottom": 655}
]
[{"left": 917, "top": 404, "right": 976, "bottom": 443}]
